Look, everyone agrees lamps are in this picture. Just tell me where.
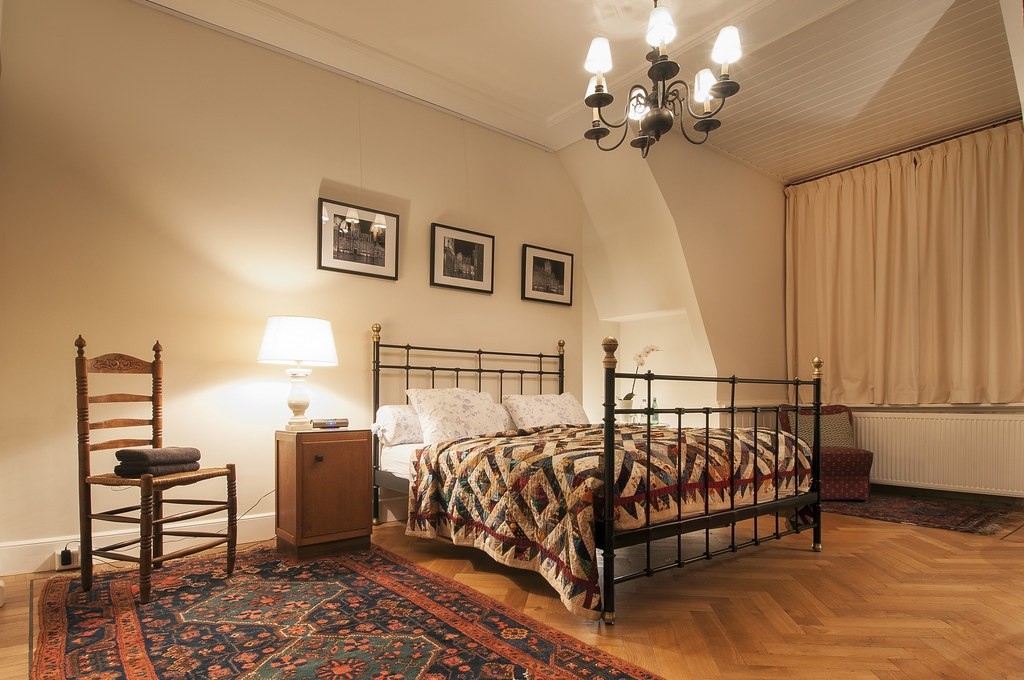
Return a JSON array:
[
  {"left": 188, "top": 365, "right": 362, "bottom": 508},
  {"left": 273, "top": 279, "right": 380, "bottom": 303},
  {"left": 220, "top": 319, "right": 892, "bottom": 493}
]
[
  {"left": 257, "top": 315, "right": 340, "bottom": 432},
  {"left": 583, "top": 0, "right": 743, "bottom": 158}
]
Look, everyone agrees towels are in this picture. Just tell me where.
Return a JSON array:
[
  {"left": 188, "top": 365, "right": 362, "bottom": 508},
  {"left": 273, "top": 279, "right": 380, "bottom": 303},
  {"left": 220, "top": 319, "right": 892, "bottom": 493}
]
[
  {"left": 113, "top": 446, "right": 202, "bottom": 466},
  {"left": 114, "top": 462, "right": 200, "bottom": 479}
]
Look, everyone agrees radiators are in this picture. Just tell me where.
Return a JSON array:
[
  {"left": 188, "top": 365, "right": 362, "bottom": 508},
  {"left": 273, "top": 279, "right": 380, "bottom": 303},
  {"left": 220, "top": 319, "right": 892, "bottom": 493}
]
[{"left": 851, "top": 411, "right": 1023, "bottom": 499}]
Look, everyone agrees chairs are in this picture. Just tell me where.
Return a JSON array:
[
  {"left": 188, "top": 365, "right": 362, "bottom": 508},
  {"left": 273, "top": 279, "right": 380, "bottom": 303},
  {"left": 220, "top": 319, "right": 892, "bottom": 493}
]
[{"left": 75, "top": 335, "right": 237, "bottom": 604}]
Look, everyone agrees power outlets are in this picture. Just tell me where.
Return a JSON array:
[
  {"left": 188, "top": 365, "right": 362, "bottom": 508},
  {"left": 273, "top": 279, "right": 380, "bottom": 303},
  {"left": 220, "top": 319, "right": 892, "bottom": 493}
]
[{"left": 55, "top": 548, "right": 77, "bottom": 572}]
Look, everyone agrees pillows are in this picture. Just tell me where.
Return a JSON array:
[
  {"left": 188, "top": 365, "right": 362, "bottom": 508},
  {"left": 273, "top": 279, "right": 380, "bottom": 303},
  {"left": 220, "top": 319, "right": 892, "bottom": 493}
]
[
  {"left": 788, "top": 411, "right": 854, "bottom": 446},
  {"left": 502, "top": 391, "right": 591, "bottom": 429},
  {"left": 371, "top": 403, "right": 518, "bottom": 446},
  {"left": 405, "top": 388, "right": 506, "bottom": 444}
]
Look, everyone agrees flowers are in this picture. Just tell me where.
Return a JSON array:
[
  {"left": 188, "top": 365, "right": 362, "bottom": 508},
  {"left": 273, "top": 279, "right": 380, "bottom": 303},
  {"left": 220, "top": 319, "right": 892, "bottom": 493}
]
[{"left": 616, "top": 344, "right": 662, "bottom": 401}]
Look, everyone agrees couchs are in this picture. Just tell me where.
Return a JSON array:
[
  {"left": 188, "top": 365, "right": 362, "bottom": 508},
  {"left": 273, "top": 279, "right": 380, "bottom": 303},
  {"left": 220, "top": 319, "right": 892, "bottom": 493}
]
[{"left": 778, "top": 403, "right": 873, "bottom": 502}]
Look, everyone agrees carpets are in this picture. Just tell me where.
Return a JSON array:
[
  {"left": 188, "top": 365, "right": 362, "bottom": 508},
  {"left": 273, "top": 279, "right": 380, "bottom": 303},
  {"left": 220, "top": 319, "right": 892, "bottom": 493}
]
[
  {"left": 27, "top": 530, "right": 666, "bottom": 679},
  {"left": 786, "top": 482, "right": 1023, "bottom": 536}
]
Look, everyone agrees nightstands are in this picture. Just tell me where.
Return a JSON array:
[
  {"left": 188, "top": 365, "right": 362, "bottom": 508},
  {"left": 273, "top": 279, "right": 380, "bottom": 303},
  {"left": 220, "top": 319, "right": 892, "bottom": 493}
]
[{"left": 275, "top": 428, "right": 374, "bottom": 561}]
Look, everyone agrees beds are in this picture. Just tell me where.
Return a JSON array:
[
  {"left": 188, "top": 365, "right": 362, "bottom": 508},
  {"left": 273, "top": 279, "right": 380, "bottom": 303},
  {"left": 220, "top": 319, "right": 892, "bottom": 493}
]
[{"left": 371, "top": 322, "right": 824, "bottom": 627}]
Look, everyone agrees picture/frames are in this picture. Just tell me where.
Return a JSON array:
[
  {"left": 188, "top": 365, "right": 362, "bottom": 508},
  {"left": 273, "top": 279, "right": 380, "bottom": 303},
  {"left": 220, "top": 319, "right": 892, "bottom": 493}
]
[
  {"left": 522, "top": 243, "right": 575, "bottom": 306},
  {"left": 616, "top": 397, "right": 633, "bottom": 422},
  {"left": 316, "top": 197, "right": 400, "bottom": 280},
  {"left": 429, "top": 223, "right": 496, "bottom": 294}
]
